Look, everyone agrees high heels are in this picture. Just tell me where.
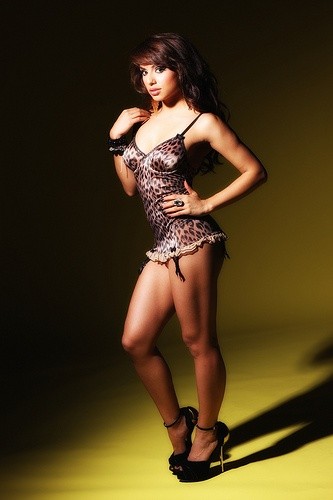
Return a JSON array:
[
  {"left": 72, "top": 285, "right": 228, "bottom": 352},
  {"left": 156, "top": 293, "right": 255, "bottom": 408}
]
[
  {"left": 163, "top": 407, "right": 199, "bottom": 468},
  {"left": 177, "top": 421, "right": 230, "bottom": 482}
]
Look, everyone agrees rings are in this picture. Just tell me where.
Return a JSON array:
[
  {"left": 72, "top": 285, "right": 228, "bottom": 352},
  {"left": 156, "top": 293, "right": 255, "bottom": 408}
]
[{"left": 172, "top": 198, "right": 183, "bottom": 207}]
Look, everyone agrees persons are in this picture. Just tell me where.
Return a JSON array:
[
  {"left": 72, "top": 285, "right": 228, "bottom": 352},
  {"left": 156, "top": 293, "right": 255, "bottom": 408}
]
[{"left": 108, "top": 26, "right": 270, "bottom": 484}]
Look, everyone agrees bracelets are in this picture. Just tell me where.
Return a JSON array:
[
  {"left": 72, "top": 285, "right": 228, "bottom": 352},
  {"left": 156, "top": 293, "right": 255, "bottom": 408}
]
[{"left": 109, "top": 136, "right": 125, "bottom": 158}]
